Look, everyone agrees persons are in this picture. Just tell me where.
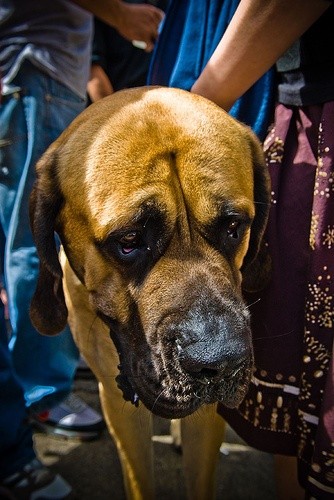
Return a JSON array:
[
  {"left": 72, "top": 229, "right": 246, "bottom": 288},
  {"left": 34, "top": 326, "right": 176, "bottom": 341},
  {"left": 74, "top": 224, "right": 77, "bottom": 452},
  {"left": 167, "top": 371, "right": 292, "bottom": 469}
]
[
  {"left": 0, "top": 296, "right": 72, "bottom": 500},
  {"left": 191, "top": 0, "right": 334, "bottom": 500},
  {"left": 0, "top": 0, "right": 172, "bottom": 437}
]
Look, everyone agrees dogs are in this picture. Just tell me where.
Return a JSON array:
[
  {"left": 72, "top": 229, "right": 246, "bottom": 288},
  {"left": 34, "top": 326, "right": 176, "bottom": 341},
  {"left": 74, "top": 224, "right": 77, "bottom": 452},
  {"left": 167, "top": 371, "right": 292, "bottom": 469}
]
[{"left": 30, "top": 85, "right": 270, "bottom": 500}]
